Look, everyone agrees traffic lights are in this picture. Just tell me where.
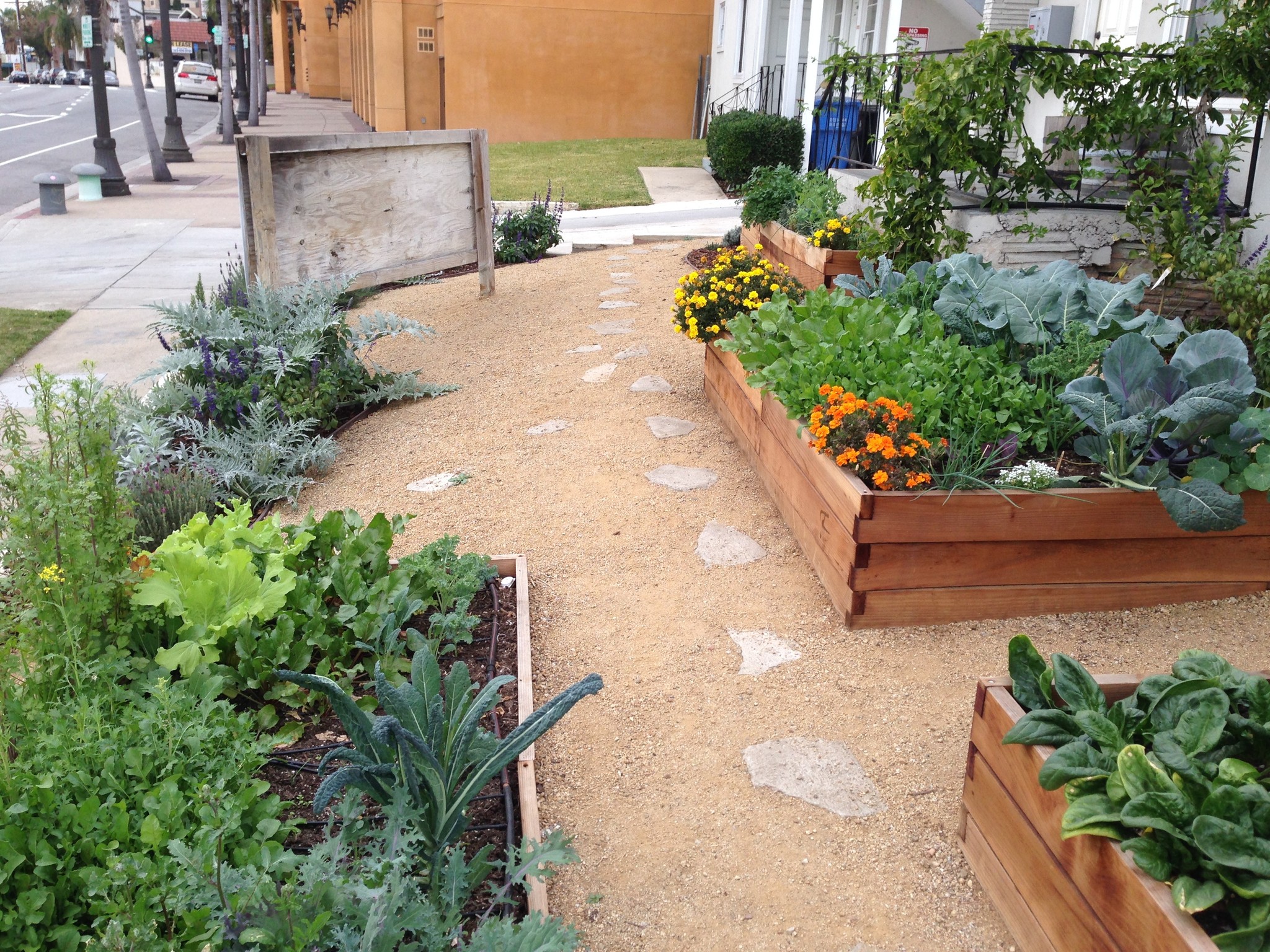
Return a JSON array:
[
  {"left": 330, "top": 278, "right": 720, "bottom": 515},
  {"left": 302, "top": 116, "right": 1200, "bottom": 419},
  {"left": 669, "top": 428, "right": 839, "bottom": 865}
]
[
  {"left": 145, "top": 26, "right": 153, "bottom": 44},
  {"left": 207, "top": 12, "right": 215, "bottom": 36}
]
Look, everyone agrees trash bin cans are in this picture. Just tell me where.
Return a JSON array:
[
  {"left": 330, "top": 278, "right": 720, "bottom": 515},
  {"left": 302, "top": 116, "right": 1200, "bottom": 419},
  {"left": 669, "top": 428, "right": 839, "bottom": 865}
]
[
  {"left": 808, "top": 96, "right": 862, "bottom": 177},
  {"left": 846, "top": 105, "right": 879, "bottom": 169}
]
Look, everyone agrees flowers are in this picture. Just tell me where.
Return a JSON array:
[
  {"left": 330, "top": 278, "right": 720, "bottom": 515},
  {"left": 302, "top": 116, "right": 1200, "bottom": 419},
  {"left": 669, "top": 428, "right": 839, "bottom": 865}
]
[{"left": 740, "top": 160, "right": 853, "bottom": 249}]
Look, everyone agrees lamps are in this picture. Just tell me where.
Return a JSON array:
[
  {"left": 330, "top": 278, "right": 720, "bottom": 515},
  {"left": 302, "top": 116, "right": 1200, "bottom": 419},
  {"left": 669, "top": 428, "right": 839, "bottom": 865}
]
[{"left": 266, "top": 0, "right": 360, "bottom": 36}]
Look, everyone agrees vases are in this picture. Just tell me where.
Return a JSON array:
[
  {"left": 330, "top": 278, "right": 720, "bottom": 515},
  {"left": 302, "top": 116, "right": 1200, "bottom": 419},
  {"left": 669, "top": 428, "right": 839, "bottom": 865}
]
[{"left": 740, "top": 202, "right": 899, "bottom": 299}]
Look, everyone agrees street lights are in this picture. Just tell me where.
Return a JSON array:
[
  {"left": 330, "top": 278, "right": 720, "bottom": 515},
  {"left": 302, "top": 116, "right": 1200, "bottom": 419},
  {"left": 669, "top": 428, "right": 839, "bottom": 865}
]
[{"left": 232, "top": 0, "right": 253, "bottom": 121}]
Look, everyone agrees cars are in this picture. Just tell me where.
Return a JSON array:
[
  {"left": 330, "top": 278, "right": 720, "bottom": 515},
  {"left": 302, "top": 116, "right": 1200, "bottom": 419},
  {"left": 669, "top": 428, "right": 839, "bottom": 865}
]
[
  {"left": 174, "top": 60, "right": 219, "bottom": 102},
  {"left": 137, "top": 49, "right": 151, "bottom": 59},
  {"left": 74, "top": 68, "right": 91, "bottom": 86},
  {"left": 30, "top": 69, "right": 44, "bottom": 84},
  {"left": 39, "top": 70, "right": 49, "bottom": 84},
  {"left": 54, "top": 69, "right": 77, "bottom": 86},
  {"left": 9, "top": 71, "right": 29, "bottom": 84},
  {"left": 46, "top": 68, "right": 62, "bottom": 85},
  {"left": 90, "top": 70, "right": 120, "bottom": 87}
]
